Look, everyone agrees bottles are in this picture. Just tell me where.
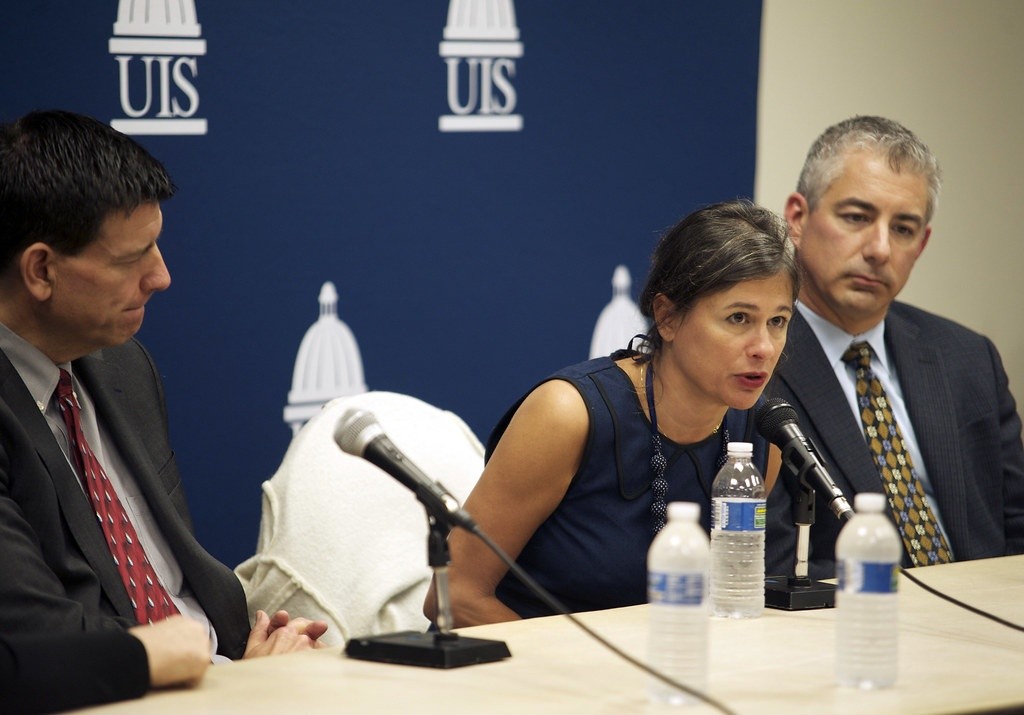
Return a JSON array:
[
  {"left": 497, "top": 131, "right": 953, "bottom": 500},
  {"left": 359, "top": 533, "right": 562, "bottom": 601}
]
[
  {"left": 834, "top": 491, "right": 900, "bottom": 692},
  {"left": 646, "top": 503, "right": 713, "bottom": 704},
  {"left": 710, "top": 442, "right": 766, "bottom": 616}
]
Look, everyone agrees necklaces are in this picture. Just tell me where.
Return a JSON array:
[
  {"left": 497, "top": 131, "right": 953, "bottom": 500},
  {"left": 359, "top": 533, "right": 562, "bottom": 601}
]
[
  {"left": 639, "top": 367, "right": 721, "bottom": 437},
  {"left": 635, "top": 346, "right": 732, "bottom": 539}
]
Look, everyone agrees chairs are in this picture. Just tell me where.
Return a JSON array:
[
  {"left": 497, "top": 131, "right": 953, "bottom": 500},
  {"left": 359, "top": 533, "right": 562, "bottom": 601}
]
[{"left": 234, "top": 391, "right": 485, "bottom": 647}]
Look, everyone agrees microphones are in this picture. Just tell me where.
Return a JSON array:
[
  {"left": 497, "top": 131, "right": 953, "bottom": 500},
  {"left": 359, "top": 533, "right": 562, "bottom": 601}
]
[
  {"left": 333, "top": 410, "right": 478, "bottom": 534},
  {"left": 757, "top": 397, "right": 856, "bottom": 523}
]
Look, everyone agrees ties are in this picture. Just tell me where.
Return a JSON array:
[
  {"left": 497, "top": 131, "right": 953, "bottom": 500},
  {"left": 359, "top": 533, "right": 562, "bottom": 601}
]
[
  {"left": 841, "top": 342, "right": 953, "bottom": 568},
  {"left": 51, "top": 367, "right": 183, "bottom": 626}
]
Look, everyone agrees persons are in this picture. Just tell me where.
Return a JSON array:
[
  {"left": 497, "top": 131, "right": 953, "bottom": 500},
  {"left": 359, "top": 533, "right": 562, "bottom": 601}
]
[
  {"left": 762, "top": 114, "right": 1024, "bottom": 579},
  {"left": 422, "top": 197, "right": 812, "bottom": 634},
  {"left": 0, "top": 107, "right": 335, "bottom": 715}
]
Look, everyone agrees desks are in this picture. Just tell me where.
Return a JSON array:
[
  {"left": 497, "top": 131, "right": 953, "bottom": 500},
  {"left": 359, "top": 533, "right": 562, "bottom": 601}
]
[{"left": 67, "top": 555, "right": 1024, "bottom": 715}]
